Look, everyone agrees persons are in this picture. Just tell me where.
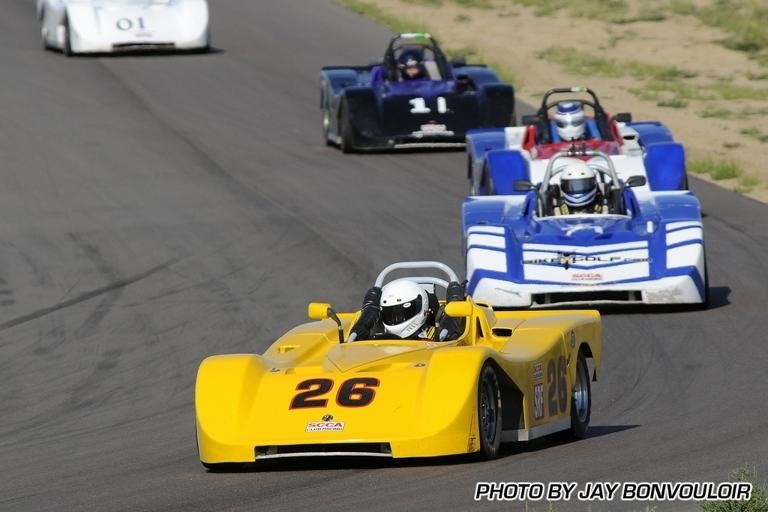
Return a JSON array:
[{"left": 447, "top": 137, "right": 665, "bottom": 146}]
[
  {"left": 552, "top": 98, "right": 589, "bottom": 142},
  {"left": 369, "top": 277, "right": 448, "bottom": 340},
  {"left": 549, "top": 164, "right": 616, "bottom": 216},
  {"left": 396, "top": 49, "right": 427, "bottom": 83}
]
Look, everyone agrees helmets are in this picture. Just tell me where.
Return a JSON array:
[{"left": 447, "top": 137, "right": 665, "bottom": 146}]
[
  {"left": 398, "top": 50, "right": 424, "bottom": 80},
  {"left": 379, "top": 279, "right": 429, "bottom": 339},
  {"left": 559, "top": 163, "right": 598, "bottom": 207},
  {"left": 554, "top": 100, "right": 586, "bottom": 141}
]
